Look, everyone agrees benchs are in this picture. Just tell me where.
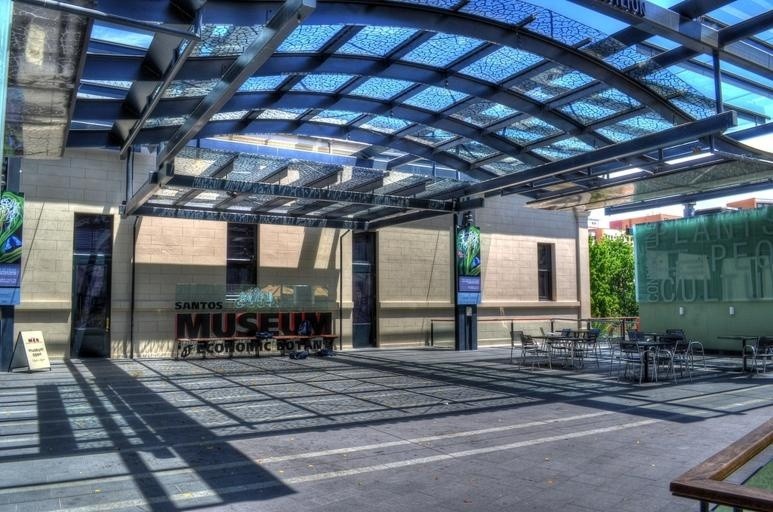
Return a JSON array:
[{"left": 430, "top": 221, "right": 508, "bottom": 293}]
[
  {"left": 273, "top": 335, "right": 338, "bottom": 356},
  {"left": 175, "top": 338, "right": 259, "bottom": 359}
]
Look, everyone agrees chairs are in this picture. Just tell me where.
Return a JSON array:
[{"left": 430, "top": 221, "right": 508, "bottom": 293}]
[{"left": 507, "top": 327, "right": 773, "bottom": 387}]
